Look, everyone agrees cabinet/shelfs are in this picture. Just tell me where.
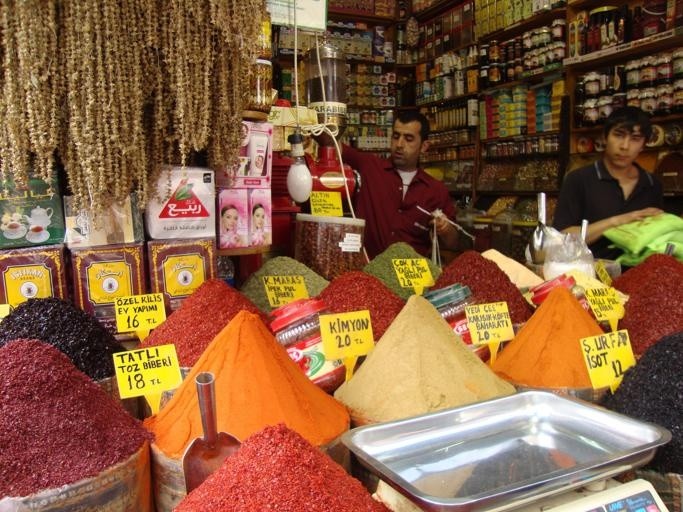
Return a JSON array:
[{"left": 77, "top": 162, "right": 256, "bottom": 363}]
[{"left": 269, "top": 1, "right": 683, "bottom": 259}]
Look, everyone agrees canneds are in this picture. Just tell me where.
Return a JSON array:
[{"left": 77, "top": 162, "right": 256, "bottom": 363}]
[
  {"left": 245, "top": 59, "right": 273, "bottom": 113},
  {"left": 479, "top": 18, "right": 683, "bottom": 157}
]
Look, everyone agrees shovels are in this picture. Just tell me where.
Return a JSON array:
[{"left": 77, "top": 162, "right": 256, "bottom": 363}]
[
  {"left": 528, "top": 193, "right": 557, "bottom": 265},
  {"left": 182, "top": 371, "right": 243, "bottom": 495}
]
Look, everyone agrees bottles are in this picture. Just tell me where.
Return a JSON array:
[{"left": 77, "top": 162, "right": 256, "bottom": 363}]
[
  {"left": 396, "top": 24, "right": 404, "bottom": 64},
  {"left": 580, "top": 3, "right": 641, "bottom": 56}
]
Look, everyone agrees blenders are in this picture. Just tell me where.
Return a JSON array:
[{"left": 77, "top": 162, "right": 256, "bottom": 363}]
[{"left": 305, "top": 42, "right": 363, "bottom": 202}]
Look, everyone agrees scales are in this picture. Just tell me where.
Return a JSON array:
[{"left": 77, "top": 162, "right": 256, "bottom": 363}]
[{"left": 339, "top": 390, "right": 672, "bottom": 512}]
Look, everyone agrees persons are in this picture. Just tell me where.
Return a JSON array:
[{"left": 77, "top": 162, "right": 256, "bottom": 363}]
[
  {"left": 220, "top": 203, "right": 242, "bottom": 247},
  {"left": 337, "top": 105, "right": 464, "bottom": 263},
  {"left": 246, "top": 204, "right": 270, "bottom": 246},
  {"left": 553, "top": 104, "right": 664, "bottom": 263}
]
